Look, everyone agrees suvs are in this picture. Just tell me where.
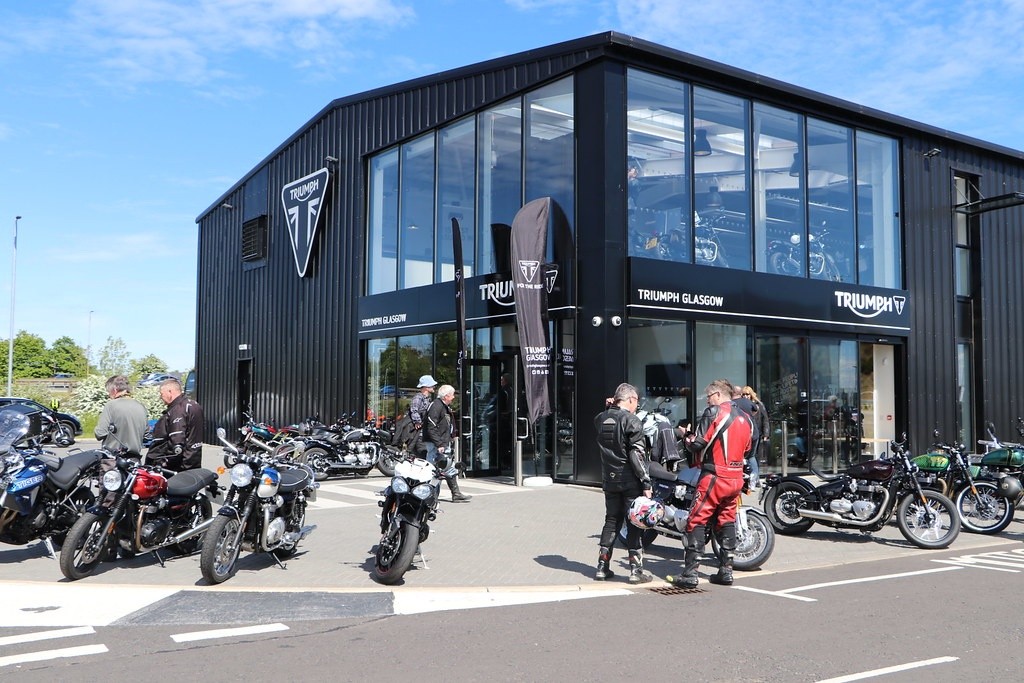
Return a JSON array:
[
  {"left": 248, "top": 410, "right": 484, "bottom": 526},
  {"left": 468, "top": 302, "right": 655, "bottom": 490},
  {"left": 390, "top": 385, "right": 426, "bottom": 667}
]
[{"left": 0, "top": 397, "right": 82, "bottom": 449}]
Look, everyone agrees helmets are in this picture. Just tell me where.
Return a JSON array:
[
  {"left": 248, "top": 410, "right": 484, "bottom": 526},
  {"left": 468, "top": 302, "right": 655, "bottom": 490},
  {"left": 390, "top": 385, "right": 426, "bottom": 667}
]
[
  {"left": 627, "top": 496, "right": 665, "bottom": 529},
  {"left": 998, "top": 476, "right": 1023, "bottom": 499},
  {"left": 298, "top": 421, "right": 313, "bottom": 435}
]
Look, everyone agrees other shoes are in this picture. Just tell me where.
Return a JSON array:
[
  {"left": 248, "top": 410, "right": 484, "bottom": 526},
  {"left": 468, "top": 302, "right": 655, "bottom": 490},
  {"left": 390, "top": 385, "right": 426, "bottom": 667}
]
[
  {"left": 750, "top": 474, "right": 757, "bottom": 492},
  {"left": 757, "top": 480, "right": 761, "bottom": 488}
]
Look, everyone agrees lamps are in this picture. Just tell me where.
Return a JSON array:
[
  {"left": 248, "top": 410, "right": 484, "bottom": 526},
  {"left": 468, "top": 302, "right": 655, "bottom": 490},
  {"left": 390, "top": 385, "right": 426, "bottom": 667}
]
[
  {"left": 223, "top": 204, "right": 233, "bottom": 209},
  {"left": 925, "top": 148, "right": 941, "bottom": 156},
  {"left": 691, "top": 129, "right": 713, "bottom": 156},
  {"left": 789, "top": 153, "right": 810, "bottom": 177},
  {"left": 325, "top": 155, "right": 338, "bottom": 173}
]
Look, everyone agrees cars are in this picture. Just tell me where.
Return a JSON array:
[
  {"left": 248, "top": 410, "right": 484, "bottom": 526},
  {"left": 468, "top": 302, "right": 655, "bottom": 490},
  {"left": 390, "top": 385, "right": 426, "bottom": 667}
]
[
  {"left": 379, "top": 386, "right": 406, "bottom": 399},
  {"left": 138, "top": 374, "right": 182, "bottom": 389},
  {"left": 54, "top": 374, "right": 77, "bottom": 388}
]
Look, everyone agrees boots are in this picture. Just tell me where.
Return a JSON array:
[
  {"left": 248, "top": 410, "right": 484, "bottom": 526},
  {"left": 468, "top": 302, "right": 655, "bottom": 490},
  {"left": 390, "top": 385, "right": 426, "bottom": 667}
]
[
  {"left": 666, "top": 527, "right": 705, "bottom": 589},
  {"left": 629, "top": 549, "right": 653, "bottom": 584},
  {"left": 446, "top": 476, "right": 472, "bottom": 503},
  {"left": 710, "top": 526, "right": 735, "bottom": 585},
  {"left": 595, "top": 547, "right": 614, "bottom": 581}
]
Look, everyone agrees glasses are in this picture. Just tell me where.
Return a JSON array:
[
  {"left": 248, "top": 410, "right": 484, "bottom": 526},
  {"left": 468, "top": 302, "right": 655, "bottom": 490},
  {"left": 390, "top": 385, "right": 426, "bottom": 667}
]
[
  {"left": 742, "top": 393, "right": 749, "bottom": 395},
  {"left": 706, "top": 392, "right": 717, "bottom": 399}
]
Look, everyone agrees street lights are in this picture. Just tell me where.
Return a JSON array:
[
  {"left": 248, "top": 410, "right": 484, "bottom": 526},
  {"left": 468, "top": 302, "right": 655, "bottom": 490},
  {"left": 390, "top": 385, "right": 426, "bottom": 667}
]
[
  {"left": 87, "top": 311, "right": 94, "bottom": 379},
  {"left": 7, "top": 216, "right": 23, "bottom": 394}
]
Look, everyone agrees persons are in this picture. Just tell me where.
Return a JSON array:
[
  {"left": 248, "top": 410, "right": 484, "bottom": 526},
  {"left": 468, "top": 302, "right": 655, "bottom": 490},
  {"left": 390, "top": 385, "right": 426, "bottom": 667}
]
[
  {"left": 595, "top": 382, "right": 654, "bottom": 584},
  {"left": 95, "top": 375, "right": 148, "bottom": 563},
  {"left": 732, "top": 386, "right": 770, "bottom": 491},
  {"left": 820, "top": 392, "right": 858, "bottom": 468},
  {"left": 666, "top": 380, "right": 753, "bottom": 587},
  {"left": 495, "top": 371, "right": 514, "bottom": 470},
  {"left": 406, "top": 375, "right": 437, "bottom": 461},
  {"left": 424, "top": 384, "right": 473, "bottom": 503},
  {"left": 144, "top": 379, "right": 203, "bottom": 475}
]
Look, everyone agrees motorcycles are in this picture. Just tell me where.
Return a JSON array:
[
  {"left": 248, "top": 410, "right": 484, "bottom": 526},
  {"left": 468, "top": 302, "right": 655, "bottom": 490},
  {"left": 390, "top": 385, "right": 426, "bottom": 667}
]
[
  {"left": 759, "top": 431, "right": 961, "bottom": 550},
  {"left": 0, "top": 409, "right": 112, "bottom": 563},
  {"left": 200, "top": 427, "right": 320, "bottom": 584},
  {"left": 367, "top": 441, "right": 467, "bottom": 584},
  {"left": 644, "top": 204, "right": 731, "bottom": 268},
  {"left": 635, "top": 397, "right": 672, "bottom": 469},
  {"left": 764, "top": 398, "right": 858, "bottom": 465},
  {"left": 224, "top": 403, "right": 402, "bottom": 478},
  {"left": 59, "top": 422, "right": 227, "bottom": 580},
  {"left": 767, "top": 220, "right": 840, "bottom": 282},
  {"left": 896, "top": 420, "right": 1024, "bottom": 535},
  {"left": 618, "top": 455, "right": 776, "bottom": 571}
]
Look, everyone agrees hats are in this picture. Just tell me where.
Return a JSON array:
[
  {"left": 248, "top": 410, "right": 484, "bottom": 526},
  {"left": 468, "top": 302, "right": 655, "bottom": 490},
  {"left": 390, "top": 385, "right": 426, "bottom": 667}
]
[{"left": 417, "top": 375, "right": 437, "bottom": 388}]
